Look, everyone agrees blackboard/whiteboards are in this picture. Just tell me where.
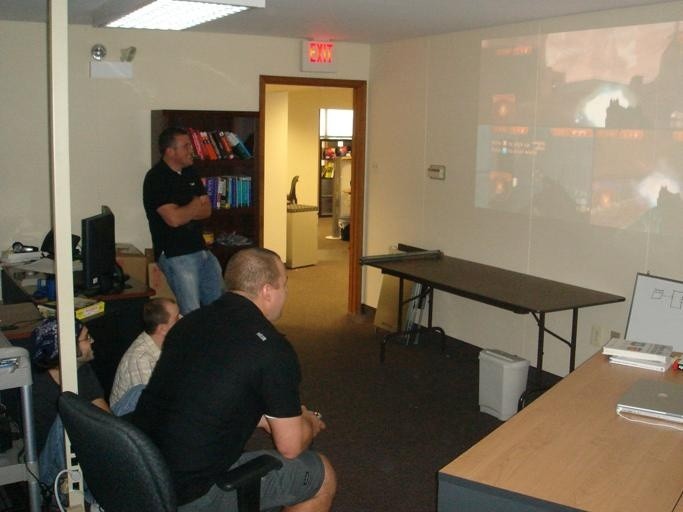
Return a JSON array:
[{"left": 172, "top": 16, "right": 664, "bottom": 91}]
[{"left": 624, "top": 273, "right": 683, "bottom": 353}]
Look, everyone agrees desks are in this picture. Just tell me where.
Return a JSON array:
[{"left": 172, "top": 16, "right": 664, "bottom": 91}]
[
  {"left": 361, "top": 251, "right": 627, "bottom": 412},
  {"left": 0, "top": 299, "right": 47, "bottom": 341},
  {"left": 438, "top": 338, "right": 682, "bottom": 512},
  {"left": 1, "top": 251, "right": 154, "bottom": 303}
]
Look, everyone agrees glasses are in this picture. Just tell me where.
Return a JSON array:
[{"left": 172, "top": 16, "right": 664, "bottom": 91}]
[{"left": 79, "top": 334, "right": 93, "bottom": 345}]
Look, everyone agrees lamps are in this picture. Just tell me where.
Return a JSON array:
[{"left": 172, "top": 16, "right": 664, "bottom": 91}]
[
  {"left": 89, "top": 44, "right": 136, "bottom": 80},
  {"left": 92, "top": 0, "right": 267, "bottom": 32}
]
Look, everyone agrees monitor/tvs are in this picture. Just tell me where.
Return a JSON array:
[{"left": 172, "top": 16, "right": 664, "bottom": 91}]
[
  {"left": 82, "top": 214, "right": 126, "bottom": 297},
  {"left": 101, "top": 205, "right": 131, "bottom": 284}
]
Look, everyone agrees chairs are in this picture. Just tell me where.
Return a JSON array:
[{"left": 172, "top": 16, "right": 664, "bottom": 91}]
[{"left": 58, "top": 391, "right": 284, "bottom": 512}]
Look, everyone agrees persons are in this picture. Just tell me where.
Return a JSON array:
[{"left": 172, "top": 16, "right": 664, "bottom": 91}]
[
  {"left": 110, "top": 297, "right": 180, "bottom": 418},
  {"left": 134, "top": 247, "right": 337, "bottom": 512},
  {"left": 31, "top": 318, "right": 109, "bottom": 498},
  {"left": 142, "top": 126, "right": 223, "bottom": 317}
]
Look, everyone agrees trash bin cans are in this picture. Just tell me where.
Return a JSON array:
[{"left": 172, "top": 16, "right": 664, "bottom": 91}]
[
  {"left": 479, "top": 348, "right": 530, "bottom": 422},
  {"left": 338, "top": 218, "right": 350, "bottom": 241}
]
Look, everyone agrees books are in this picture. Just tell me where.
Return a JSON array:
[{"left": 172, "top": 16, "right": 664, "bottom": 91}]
[
  {"left": 188, "top": 129, "right": 252, "bottom": 160},
  {"left": 198, "top": 174, "right": 254, "bottom": 210},
  {"left": 602, "top": 337, "right": 678, "bottom": 373}
]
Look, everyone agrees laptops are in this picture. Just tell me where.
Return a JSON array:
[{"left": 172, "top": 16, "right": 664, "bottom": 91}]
[{"left": 615, "top": 378, "right": 683, "bottom": 423}]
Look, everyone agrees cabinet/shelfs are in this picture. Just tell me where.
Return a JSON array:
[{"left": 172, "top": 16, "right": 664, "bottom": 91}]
[
  {"left": 151, "top": 109, "right": 261, "bottom": 276},
  {"left": 332, "top": 157, "right": 353, "bottom": 238},
  {"left": 287, "top": 204, "right": 319, "bottom": 269},
  {"left": 0, "top": 342, "right": 45, "bottom": 512},
  {"left": 320, "top": 137, "right": 352, "bottom": 216}
]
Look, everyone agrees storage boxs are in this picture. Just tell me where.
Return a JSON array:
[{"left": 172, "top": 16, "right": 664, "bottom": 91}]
[
  {"left": 146, "top": 261, "right": 179, "bottom": 302},
  {"left": 115, "top": 243, "right": 147, "bottom": 287}
]
